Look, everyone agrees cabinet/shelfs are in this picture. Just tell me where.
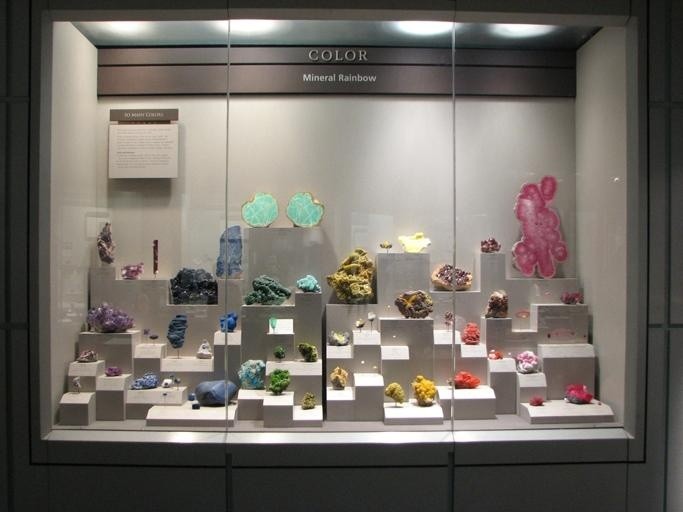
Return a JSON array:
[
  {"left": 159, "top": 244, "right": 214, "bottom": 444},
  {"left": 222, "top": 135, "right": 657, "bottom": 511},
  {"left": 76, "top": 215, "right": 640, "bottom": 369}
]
[{"left": 30, "top": 4, "right": 649, "bottom": 465}]
